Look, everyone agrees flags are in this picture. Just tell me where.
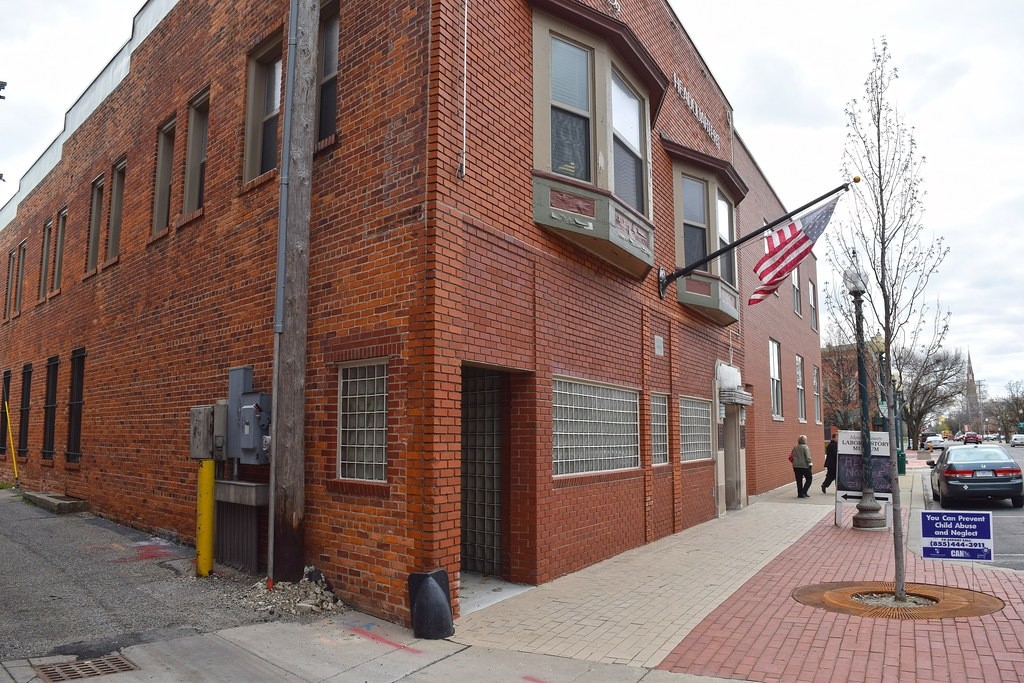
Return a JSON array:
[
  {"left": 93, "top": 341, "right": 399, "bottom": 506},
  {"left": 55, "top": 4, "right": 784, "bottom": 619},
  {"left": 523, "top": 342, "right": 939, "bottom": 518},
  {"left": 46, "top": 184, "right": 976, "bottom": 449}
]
[{"left": 748, "top": 198, "right": 840, "bottom": 305}]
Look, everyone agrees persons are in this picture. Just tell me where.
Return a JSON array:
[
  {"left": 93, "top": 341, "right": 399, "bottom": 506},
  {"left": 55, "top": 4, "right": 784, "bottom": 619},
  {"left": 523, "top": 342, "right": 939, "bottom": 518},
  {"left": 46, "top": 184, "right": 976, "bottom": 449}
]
[
  {"left": 820, "top": 434, "right": 837, "bottom": 493},
  {"left": 792, "top": 435, "right": 812, "bottom": 498}
]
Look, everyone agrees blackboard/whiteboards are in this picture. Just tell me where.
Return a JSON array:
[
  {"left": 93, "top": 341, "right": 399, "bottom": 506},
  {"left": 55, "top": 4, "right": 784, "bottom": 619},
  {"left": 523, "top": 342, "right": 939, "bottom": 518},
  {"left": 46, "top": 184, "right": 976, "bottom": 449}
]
[{"left": 835, "top": 453, "right": 893, "bottom": 493}]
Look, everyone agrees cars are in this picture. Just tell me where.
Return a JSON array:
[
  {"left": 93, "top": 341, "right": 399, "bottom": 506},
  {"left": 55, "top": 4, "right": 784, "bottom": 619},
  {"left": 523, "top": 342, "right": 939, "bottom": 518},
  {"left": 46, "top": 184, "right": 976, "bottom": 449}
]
[
  {"left": 924, "top": 436, "right": 945, "bottom": 452},
  {"left": 980, "top": 434, "right": 1005, "bottom": 441},
  {"left": 925, "top": 444, "right": 1024, "bottom": 510},
  {"left": 1010, "top": 434, "right": 1024, "bottom": 447}
]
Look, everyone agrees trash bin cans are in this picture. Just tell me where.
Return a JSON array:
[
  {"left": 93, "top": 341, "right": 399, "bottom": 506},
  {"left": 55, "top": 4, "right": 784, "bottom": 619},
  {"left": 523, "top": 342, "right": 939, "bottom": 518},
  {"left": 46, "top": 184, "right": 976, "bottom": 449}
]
[{"left": 897, "top": 451, "right": 908, "bottom": 477}]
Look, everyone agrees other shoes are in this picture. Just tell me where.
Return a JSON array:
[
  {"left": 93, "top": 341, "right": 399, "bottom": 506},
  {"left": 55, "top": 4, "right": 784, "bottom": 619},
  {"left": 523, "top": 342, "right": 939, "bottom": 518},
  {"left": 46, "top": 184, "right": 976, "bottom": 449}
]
[
  {"left": 798, "top": 495, "right": 804, "bottom": 498},
  {"left": 821, "top": 483, "right": 826, "bottom": 494},
  {"left": 802, "top": 491, "right": 809, "bottom": 497}
]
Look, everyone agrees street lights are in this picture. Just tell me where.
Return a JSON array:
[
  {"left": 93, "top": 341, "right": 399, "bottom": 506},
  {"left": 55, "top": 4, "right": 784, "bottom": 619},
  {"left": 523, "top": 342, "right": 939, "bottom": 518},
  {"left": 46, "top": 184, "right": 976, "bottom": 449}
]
[
  {"left": 870, "top": 327, "right": 889, "bottom": 433},
  {"left": 843, "top": 248, "right": 888, "bottom": 529}
]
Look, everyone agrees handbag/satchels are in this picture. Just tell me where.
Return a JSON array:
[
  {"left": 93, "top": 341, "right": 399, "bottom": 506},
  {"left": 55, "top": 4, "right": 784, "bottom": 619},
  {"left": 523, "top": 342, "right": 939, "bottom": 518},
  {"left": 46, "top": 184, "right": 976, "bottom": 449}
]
[{"left": 788, "top": 446, "right": 794, "bottom": 463}]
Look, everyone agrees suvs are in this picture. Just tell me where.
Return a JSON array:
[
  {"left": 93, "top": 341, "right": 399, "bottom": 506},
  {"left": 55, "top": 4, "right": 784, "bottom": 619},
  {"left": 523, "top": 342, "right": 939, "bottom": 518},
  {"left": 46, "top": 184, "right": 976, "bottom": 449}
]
[
  {"left": 920, "top": 432, "right": 937, "bottom": 448},
  {"left": 953, "top": 432, "right": 982, "bottom": 444}
]
